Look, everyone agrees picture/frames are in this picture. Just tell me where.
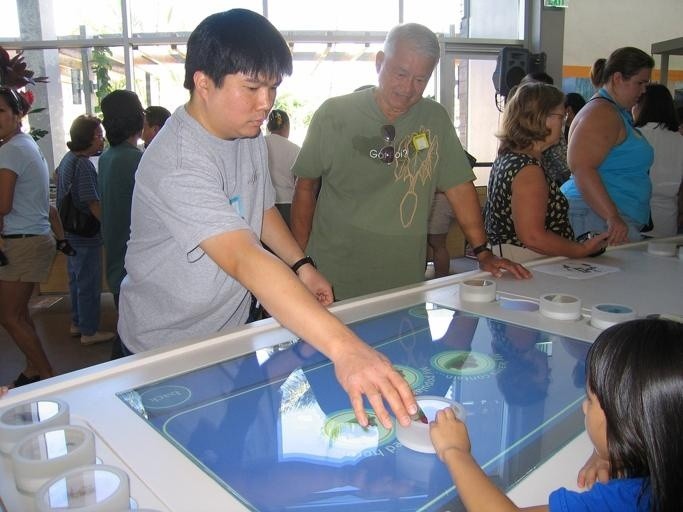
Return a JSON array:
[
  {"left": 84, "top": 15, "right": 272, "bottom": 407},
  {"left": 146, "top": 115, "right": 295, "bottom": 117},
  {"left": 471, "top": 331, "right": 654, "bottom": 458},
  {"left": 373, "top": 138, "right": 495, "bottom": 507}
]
[{"left": 1, "top": 223, "right": 683, "bottom": 512}]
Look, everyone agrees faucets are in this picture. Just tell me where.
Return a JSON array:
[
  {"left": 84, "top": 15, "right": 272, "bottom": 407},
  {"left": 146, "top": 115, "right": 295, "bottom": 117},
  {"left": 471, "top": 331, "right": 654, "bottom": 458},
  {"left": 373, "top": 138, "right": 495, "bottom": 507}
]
[{"left": 53, "top": 238, "right": 77, "bottom": 256}]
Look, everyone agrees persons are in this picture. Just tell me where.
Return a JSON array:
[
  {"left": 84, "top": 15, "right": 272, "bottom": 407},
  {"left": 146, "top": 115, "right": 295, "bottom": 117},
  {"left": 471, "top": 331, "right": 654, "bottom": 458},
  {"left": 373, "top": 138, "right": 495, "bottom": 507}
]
[
  {"left": 296, "top": 302, "right": 479, "bottom": 420},
  {"left": 484, "top": 46, "right": 683, "bottom": 258},
  {"left": 485, "top": 316, "right": 551, "bottom": 408},
  {"left": 1, "top": 86, "right": 172, "bottom": 389},
  {"left": 560, "top": 335, "right": 592, "bottom": 388},
  {"left": 148, "top": 339, "right": 416, "bottom": 512},
  {"left": 429, "top": 318, "right": 683, "bottom": 512},
  {"left": 424, "top": 188, "right": 454, "bottom": 277},
  {"left": 117, "top": 12, "right": 419, "bottom": 429},
  {"left": 430, "top": 457, "right": 465, "bottom": 511},
  {"left": 265, "top": 109, "right": 301, "bottom": 231},
  {"left": 290, "top": 23, "right": 532, "bottom": 302}
]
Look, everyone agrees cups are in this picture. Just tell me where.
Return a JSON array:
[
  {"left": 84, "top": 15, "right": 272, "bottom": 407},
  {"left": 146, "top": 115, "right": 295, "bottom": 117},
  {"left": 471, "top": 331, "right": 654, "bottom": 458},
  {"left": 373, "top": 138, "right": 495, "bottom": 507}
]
[
  {"left": 379, "top": 122, "right": 396, "bottom": 165},
  {"left": 0, "top": 88, "right": 20, "bottom": 105},
  {"left": 545, "top": 110, "right": 569, "bottom": 122}
]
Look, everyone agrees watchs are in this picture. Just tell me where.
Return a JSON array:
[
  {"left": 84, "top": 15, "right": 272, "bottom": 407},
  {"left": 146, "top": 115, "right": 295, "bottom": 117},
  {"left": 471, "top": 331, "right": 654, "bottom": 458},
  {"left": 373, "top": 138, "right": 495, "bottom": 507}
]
[
  {"left": 474, "top": 241, "right": 489, "bottom": 257},
  {"left": 289, "top": 255, "right": 317, "bottom": 274}
]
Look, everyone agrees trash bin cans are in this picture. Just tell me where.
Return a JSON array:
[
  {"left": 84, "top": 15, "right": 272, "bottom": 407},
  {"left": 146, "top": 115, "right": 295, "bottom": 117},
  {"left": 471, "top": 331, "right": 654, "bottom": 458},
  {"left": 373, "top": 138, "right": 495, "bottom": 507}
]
[
  {"left": 289, "top": 255, "right": 317, "bottom": 274},
  {"left": 474, "top": 241, "right": 489, "bottom": 257}
]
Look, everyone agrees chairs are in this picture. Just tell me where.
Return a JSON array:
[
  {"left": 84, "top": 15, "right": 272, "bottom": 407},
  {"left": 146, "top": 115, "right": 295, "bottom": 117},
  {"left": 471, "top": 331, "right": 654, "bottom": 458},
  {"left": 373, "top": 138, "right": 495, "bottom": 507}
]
[{"left": 68, "top": 322, "right": 114, "bottom": 345}]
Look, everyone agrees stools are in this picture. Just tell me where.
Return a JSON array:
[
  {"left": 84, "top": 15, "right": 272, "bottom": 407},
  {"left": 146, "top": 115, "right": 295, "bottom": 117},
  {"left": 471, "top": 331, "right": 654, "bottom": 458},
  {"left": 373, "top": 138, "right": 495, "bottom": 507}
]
[{"left": 2, "top": 233, "right": 40, "bottom": 238}]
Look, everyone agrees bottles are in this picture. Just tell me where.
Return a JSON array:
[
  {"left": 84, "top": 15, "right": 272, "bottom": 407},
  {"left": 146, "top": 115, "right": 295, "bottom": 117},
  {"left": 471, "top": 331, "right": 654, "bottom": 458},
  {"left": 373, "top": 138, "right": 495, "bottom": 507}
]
[{"left": 101, "top": 89, "right": 147, "bottom": 117}]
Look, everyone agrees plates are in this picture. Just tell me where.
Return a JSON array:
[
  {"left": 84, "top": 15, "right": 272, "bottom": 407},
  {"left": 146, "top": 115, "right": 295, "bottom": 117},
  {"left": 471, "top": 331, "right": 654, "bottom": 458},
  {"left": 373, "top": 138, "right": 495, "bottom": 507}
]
[
  {"left": 651, "top": 36, "right": 683, "bottom": 87},
  {"left": 427, "top": 222, "right": 466, "bottom": 262},
  {"left": 38, "top": 241, "right": 111, "bottom": 295}
]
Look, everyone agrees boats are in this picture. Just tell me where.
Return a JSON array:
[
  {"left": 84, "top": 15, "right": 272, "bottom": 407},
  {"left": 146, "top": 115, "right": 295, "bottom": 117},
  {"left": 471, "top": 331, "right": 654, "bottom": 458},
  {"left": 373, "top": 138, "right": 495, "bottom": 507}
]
[{"left": 492, "top": 47, "right": 530, "bottom": 95}]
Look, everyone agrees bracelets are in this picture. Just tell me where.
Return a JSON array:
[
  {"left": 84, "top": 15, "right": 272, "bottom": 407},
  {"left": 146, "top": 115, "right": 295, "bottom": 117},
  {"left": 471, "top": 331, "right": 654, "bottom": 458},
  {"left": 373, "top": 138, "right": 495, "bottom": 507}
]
[{"left": 289, "top": 342, "right": 307, "bottom": 361}]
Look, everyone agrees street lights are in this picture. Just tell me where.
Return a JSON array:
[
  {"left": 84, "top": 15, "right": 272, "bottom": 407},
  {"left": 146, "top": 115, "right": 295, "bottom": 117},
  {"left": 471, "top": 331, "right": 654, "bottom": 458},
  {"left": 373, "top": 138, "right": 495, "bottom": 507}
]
[{"left": 56, "top": 191, "right": 101, "bottom": 236}]
